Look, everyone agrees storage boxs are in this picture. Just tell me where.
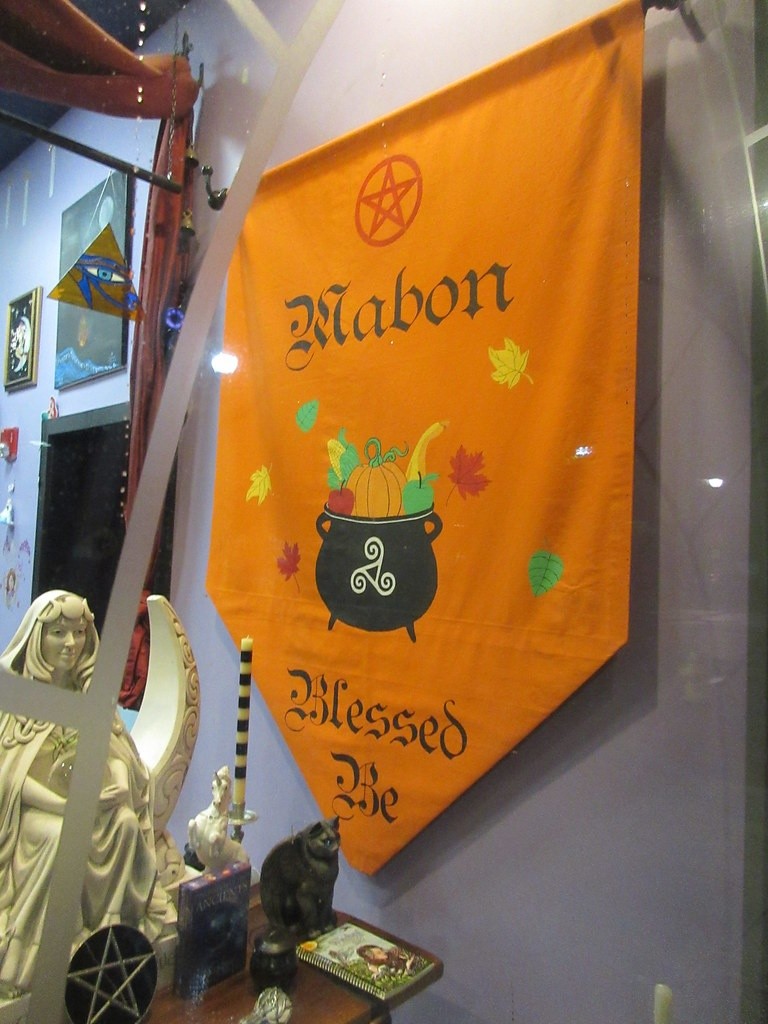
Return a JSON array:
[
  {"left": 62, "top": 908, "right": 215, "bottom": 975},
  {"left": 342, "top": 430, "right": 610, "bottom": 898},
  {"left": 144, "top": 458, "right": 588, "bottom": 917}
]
[{"left": 175, "top": 861, "right": 252, "bottom": 998}]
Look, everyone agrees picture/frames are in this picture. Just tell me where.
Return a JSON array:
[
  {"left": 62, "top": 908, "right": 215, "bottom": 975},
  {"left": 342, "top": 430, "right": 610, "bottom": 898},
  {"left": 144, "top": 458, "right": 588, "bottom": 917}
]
[{"left": 3, "top": 286, "right": 42, "bottom": 392}]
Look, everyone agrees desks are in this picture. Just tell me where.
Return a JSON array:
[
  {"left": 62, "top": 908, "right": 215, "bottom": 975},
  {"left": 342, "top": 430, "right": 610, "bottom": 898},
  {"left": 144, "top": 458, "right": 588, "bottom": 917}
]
[{"left": 147, "top": 878, "right": 444, "bottom": 1024}]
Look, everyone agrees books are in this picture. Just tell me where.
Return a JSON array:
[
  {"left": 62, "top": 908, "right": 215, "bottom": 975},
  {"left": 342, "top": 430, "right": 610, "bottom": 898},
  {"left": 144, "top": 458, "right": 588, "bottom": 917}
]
[{"left": 295, "top": 920, "right": 434, "bottom": 1001}]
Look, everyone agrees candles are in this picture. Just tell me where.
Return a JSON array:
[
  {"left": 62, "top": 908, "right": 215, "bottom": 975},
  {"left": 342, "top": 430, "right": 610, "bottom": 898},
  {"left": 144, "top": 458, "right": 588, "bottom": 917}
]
[{"left": 233, "top": 634, "right": 253, "bottom": 804}]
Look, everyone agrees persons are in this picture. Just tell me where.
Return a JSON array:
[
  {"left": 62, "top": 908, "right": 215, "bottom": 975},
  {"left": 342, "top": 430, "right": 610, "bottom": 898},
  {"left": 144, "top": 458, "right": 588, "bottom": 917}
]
[{"left": 1, "top": 588, "right": 176, "bottom": 995}]
